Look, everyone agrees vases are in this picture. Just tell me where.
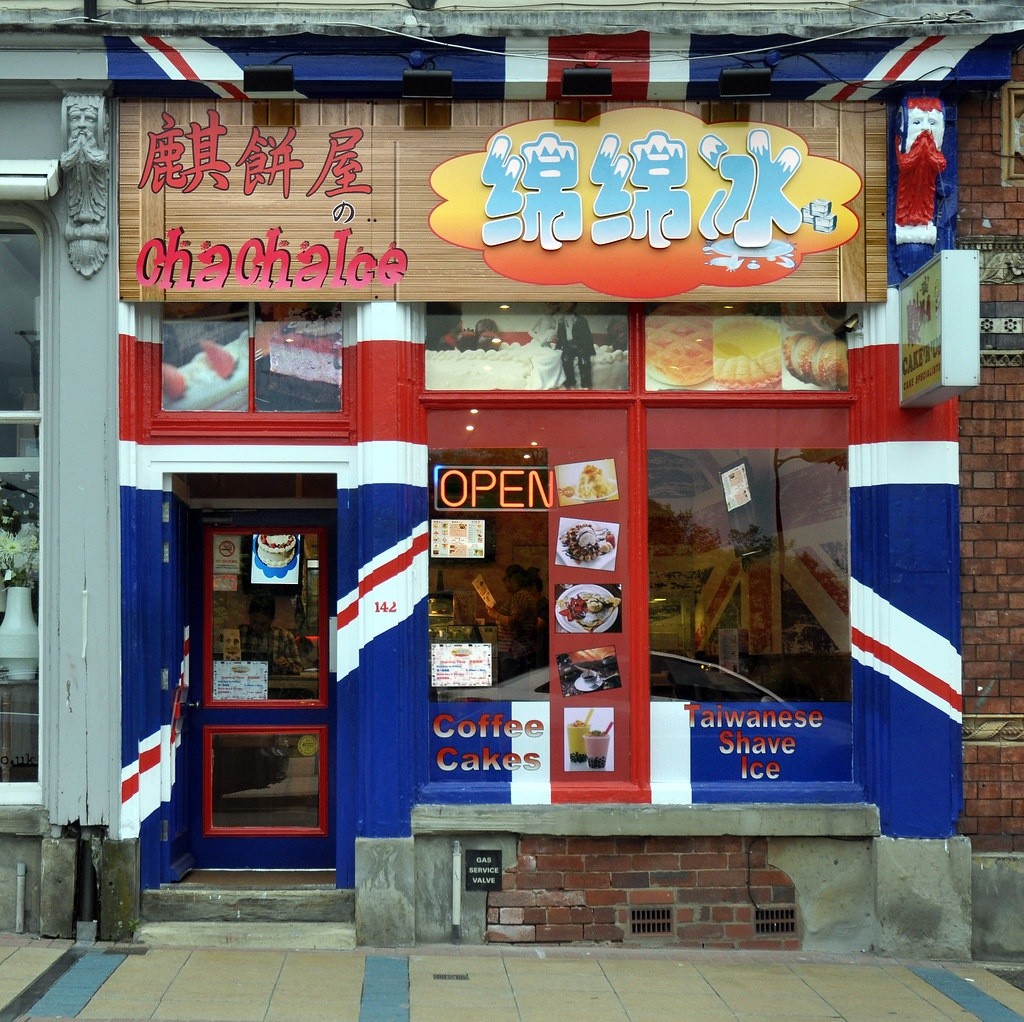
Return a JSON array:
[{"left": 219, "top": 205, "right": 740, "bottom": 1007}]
[{"left": 0, "top": 586, "right": 39, "bottom": 681}]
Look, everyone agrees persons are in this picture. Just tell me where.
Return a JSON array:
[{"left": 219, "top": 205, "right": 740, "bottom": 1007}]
[
  {"left": 522, "top": 565, "right": 552, "bottom": 669},
  {"left": 485, "top": 563, "right": 539, "bottom": 683}
]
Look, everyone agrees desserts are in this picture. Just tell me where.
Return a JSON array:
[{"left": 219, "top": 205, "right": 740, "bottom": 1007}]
[{"left": 156, "top": 303, "right": 344, "bottom": 415}]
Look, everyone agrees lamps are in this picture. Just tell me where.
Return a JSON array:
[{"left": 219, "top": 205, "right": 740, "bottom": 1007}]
[
  {"left": 718, "top": 62, "right": 773, "bottom": 101},
  {"left": 401, "top": 58, "right": 452, "bottom": 100},
  {"left": 242, "top": 64, "right": 295, "bottom": 92},
  {"left": 561, "top": 64, "right": 613, "bottom": 97}
]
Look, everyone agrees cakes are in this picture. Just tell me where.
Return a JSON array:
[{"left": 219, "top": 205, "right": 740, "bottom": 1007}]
[{"left": 425, "top": 304, "right": 629, "bottom": 393}]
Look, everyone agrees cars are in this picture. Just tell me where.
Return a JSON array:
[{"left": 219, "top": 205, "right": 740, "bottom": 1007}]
[{"left": 450, "top": 650, "right": 788, "bottom": 707}]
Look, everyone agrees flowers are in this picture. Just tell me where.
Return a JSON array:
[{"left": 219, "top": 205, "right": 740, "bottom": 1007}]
[{"left": 0, "top": 522, "right": 40, "bottom": 589}]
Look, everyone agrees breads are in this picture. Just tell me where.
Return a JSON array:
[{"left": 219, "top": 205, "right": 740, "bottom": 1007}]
[
  {"left": 561, "top": 525, "right": 616, "bottom": 562},
  {"left": 644, "top": 303, "right": 850, "bottom": 391}
]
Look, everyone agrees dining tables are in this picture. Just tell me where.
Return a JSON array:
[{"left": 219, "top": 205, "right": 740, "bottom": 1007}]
[{"left": 652, "top": 668, "right": 793, "bottom": 701}]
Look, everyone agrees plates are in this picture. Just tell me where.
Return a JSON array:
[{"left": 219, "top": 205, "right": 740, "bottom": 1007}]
[
  {"left": 569, "top": 479, "right": 619, "bottom": 502},
  {"left": 574, "top": 676, "right": 604, "bottom": 693},
  {"left": 554, "top": 584, "right": 618, "bottom": 633},
  {"left": 557, "top": 531, "right": 616, "bottom": 569}
]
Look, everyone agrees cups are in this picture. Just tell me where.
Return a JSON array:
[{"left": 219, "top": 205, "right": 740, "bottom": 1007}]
[
  {"left": 568, "top": 725, "right": 590, "bottom": 763},
  {"left": 581, "top": 670, "right": 603, "bottom": 686},
  {"left": 582, "top": 734, "right": 611, "bottom": 770}
]
